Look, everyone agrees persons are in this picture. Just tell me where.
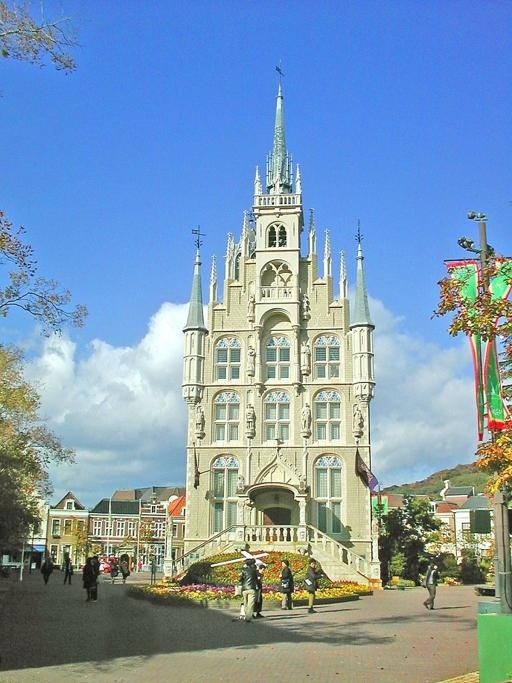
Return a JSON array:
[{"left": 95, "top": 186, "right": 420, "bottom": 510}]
[
  {"left": 278, "top": 558, "right": 295, "bottom": 610},
  {"left": 80, "top": 555, "right": 143, "bottom": 602},
  {"left": 238, "top": 557, "right": 263, "bottom": 622},
  {"left": 41, "top": 555, "right": 54, "bottom": 585},
  {"left": 303, "top": 557, "right": 325, "bottom": 614},
  {"left": 422, "top": 564, "right": 440, "bottom": 610},
  {"left": 236, "top": 558, "right": 257, "bottom": 620},
  {"left": 62, "top": 557, "right": 73, "bottom": 584},
  {"left": 252, "top": 563, "right": 266, "bottom": 617}
]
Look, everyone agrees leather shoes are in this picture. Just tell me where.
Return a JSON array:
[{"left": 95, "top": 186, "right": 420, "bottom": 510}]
[{"left": 253, "top": 614, "right": 265, "bottom": 619}]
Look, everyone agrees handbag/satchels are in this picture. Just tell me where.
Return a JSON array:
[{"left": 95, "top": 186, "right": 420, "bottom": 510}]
[{"left": 280, "top": 581, "right": 290, "bottom": 590}]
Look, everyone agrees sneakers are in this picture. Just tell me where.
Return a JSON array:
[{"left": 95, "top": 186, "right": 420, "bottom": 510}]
[
  {"left": 85, "top": 599, "right": 98, "bottom": 603},
  {"left": 423, "top": 601, "right": 429, "bottom": 610}
]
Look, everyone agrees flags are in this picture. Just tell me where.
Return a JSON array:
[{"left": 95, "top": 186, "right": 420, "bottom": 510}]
[{"left": 353, "top": 449, "right": 381, "bottom": 496}]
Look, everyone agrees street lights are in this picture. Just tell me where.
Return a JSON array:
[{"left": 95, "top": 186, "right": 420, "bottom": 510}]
[{"left": 458, "top": 210, "right": 512, "bottom": 613}]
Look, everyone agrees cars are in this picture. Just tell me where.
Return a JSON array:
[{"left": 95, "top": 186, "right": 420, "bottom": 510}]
[{"left": 98, "top": 562, "right": 110, "bottom": 573}]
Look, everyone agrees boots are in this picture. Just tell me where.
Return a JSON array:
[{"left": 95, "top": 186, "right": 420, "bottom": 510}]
[{"left": 308, "top": 605, "right": 318, "bottom": 613}]
